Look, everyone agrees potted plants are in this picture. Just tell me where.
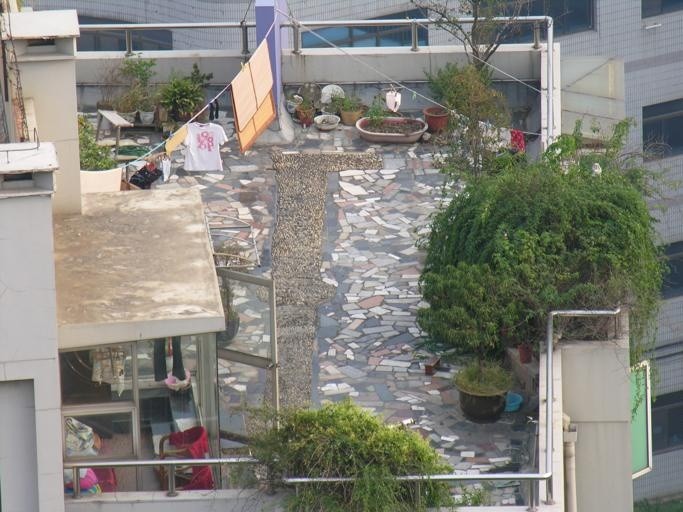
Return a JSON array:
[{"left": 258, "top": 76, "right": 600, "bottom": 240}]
[
  {"left": 320, "top": 91, "right": 362, "bottom": 126},
  {"left": 419, "top": 254, "right": 543, "bottom": 424},
  {"left": 96, "top": 52, "right": 214, "bottom": 125},
  {"left": 296, "top": 97, "right": 314, "bottom": 120},
  {"left": 215, "top": 243, "right": 252, "bottom": 343}
]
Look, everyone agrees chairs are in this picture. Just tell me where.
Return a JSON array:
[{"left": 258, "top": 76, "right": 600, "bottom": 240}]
[
  {"left": 164, "top": 465, "right": 213, "bottom": 491},
  {"left": 159, "top": 426, "right": 209, "bottom": 488}
]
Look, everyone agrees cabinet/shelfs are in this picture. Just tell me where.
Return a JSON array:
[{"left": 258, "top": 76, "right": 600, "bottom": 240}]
[{"left": 96, "top": 108, "right": 140, "bottom": 191}]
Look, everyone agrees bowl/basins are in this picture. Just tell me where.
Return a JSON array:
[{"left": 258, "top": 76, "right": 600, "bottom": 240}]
[
  {"left": 164, "top": 370, "right": 190, "bottom": 391},
  {"left": 313, "top": 114, "right": 340, "bottom": 131},
  {"left": 505, "top": 393, "right": 523, "bottom": 413}
]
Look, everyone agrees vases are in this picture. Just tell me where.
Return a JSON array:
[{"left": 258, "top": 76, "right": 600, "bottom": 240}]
[
  {"left": 422, "top": 106, "right": 449, "bottom": 133},
  {"left": 286, "top": 99, "right": 300, "bottom": 114}
]
